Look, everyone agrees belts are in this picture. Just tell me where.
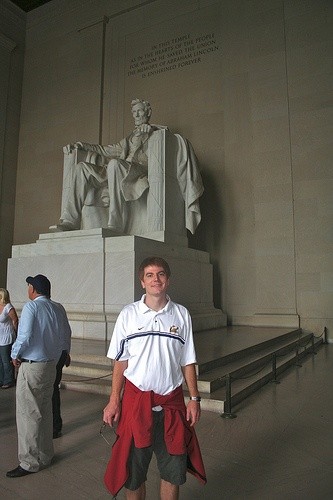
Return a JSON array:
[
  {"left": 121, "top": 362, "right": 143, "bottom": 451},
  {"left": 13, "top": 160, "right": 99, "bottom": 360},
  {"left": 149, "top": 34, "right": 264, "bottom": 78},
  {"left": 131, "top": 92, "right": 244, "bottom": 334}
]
[{"left": 20, "top": 359, "right": 37, "bottom": 363}]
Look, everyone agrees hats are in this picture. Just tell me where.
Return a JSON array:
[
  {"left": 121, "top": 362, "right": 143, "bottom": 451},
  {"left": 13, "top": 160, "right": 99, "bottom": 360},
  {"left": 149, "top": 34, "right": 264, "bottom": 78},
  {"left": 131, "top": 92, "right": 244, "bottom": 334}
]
[{"left": 26, "top": 275, "right": 51, "bottom": 293}]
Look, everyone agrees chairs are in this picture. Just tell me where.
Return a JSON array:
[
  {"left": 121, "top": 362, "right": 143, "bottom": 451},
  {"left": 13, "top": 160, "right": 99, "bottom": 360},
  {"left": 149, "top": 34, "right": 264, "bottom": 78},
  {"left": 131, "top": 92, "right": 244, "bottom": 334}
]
[{"left": 62, "top": 129, "right": 188, "bottom": 246}]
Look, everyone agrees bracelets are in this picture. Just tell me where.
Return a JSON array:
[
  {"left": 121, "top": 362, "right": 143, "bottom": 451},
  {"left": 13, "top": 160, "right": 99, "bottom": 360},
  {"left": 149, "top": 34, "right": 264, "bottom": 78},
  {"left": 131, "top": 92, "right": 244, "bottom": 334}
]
[{"left": 189, "top": 396, "right": 202, "bottom": 402}]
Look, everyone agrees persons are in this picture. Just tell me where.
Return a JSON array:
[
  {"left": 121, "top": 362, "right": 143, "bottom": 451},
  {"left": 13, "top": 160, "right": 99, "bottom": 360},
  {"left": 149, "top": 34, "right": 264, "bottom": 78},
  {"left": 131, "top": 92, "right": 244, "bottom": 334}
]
[
  {"left": 50, "top": 97, "right": 170, "bottom": 233},
  {"left": 0, "top": 274, "right": 72, "bottom": 478},
  {"left": 102, "top": 257, "right": 207, "bottom": 500}
]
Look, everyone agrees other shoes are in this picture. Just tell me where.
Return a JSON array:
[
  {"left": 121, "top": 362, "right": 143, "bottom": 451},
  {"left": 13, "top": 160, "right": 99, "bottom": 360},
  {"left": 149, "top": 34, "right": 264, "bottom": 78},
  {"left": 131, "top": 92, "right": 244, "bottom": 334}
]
[
  {"left": 2, "top": 382, "right": 15, "bottom": 389},
  {"left": 52, "top": 433, "right": 61, "bottom": 439},
  {"left": 6, "top": 465, "right": 35, "bottom": 478}
]
